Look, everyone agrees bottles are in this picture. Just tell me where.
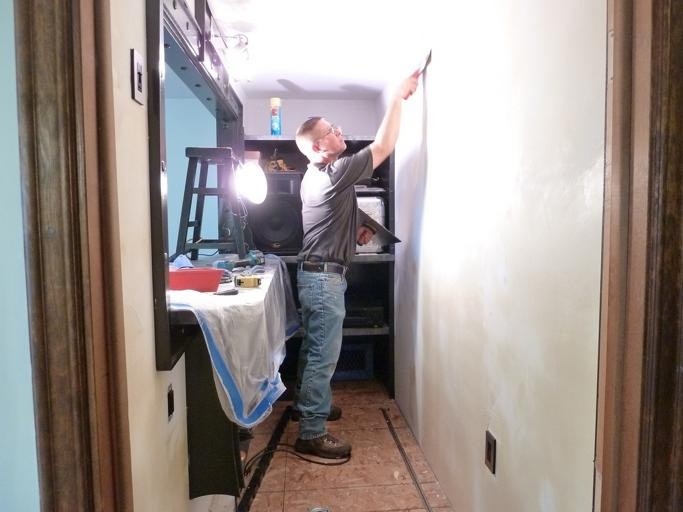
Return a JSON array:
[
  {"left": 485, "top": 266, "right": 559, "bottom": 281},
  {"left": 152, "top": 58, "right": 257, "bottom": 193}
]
[{"left": 270, "top": 97, "right": 281, "bottom": 136}]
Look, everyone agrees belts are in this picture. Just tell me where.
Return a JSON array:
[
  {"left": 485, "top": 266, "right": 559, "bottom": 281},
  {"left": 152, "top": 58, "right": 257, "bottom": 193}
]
[{"left": 299, "top": 262, "right": 343, "bottom": 275}]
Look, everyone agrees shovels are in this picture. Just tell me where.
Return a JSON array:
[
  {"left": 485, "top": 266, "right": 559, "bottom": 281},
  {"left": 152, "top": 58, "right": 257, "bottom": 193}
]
[{"left": 403, "top": 49, "right": 431, "bottom": 100}]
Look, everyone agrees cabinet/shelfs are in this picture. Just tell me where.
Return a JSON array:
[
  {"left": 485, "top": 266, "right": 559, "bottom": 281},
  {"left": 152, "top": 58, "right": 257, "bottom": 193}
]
[{"left": 283, "top": 253, "right": 394, "bottom": 401}]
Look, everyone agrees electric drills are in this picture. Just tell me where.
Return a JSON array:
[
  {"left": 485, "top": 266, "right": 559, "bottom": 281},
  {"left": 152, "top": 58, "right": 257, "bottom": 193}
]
[{"left": 213, "top": 249, "right": 265, "bottom": 271}]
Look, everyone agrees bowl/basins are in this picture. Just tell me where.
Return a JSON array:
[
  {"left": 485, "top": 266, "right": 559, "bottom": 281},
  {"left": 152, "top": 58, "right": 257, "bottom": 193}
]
[{"left": 168, "top": 267, "right": 224, "bottom": 292}]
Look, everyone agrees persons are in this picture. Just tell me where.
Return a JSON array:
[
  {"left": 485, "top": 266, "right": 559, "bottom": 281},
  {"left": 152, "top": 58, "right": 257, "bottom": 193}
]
[{"left": 291, "top": 68, "right": 420, "bottom": 466}]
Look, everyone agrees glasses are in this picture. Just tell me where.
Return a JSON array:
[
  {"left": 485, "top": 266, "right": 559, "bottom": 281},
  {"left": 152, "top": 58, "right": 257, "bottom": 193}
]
[{"left": 314, "top": 123, "right": 335, "bottom": 142}]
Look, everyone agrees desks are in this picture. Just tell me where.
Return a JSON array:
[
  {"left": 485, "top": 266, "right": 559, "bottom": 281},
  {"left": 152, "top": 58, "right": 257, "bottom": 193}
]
[{"left": 167, "top": 257, "right": 297, "bottom": 496}]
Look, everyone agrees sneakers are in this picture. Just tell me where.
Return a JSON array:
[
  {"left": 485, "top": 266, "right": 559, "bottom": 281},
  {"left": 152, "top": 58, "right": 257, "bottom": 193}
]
[
  {"left": 291, "top": 405, "right": 342, "bottom": 423},
  {"left": 294, "top": 433, "right": 352, "bottom": 459}
]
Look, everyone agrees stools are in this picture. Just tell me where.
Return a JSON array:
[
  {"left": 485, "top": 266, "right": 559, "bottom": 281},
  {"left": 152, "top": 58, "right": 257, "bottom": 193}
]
[{"left": 176, "top": 147, "right": 249, "bottom": 260}]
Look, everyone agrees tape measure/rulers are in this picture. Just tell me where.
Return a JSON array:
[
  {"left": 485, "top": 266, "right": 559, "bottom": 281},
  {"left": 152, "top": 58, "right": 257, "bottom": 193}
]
[{"left": 236, "top": 278, "right": 261, "bottom": 287}]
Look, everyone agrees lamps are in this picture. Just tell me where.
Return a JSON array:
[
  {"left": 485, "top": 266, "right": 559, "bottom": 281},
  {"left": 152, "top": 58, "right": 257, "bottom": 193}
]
[{"left": 234, "top": 151, "right": 268, "bottom": 205}]
